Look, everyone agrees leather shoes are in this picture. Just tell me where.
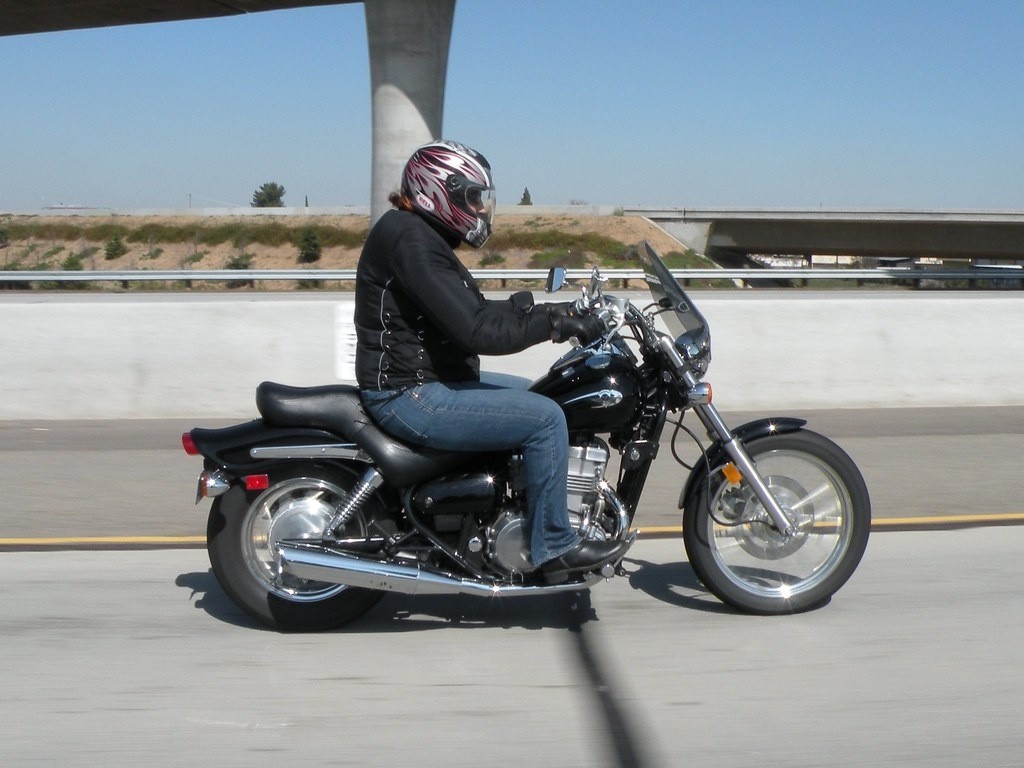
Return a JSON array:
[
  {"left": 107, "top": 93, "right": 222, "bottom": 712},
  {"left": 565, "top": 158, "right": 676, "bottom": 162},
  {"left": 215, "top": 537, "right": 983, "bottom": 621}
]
[{"left": 543, "top": 540, "right": 630, "bottom": 583}]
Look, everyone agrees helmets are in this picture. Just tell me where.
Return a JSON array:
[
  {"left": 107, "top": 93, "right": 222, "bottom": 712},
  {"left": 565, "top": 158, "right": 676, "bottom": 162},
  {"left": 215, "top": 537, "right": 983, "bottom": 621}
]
[{"left": 402, "top": 139, "right": 496, "bottom": 249}]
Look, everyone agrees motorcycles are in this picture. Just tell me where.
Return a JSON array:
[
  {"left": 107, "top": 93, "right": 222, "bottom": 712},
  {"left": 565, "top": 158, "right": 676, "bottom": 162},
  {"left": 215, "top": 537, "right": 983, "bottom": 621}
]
[{"left": 181, "top": 240, "right": 872, "bottom": 636}]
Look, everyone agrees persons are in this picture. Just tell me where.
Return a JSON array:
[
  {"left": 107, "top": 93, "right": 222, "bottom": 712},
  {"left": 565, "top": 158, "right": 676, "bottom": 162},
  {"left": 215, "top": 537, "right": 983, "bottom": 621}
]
[{"left": 354, "top": 140, "right": 631, "bottom": 587}]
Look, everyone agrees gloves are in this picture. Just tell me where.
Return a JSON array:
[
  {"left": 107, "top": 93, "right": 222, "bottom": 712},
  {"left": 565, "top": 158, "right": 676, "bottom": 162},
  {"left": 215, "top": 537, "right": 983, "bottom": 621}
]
[
  {"left": 544, "top": 302, "right": 573, "bottom": 315},
  {"left": 552, "top": 313, "right": 604, "bottom": 345}
]
